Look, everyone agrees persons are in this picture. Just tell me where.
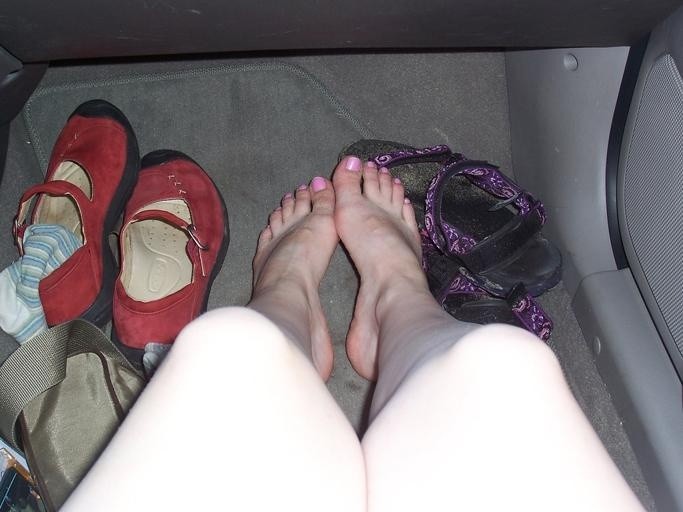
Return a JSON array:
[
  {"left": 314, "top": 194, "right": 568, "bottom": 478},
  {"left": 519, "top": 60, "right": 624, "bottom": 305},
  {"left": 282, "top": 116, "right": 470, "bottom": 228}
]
[{"left": 57, "top": 156, "right": 648, "bottom": 512}]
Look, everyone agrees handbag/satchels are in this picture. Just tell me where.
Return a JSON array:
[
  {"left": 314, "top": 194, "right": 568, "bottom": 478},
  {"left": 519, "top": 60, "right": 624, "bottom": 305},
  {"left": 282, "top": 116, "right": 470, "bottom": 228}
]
[{"left": 1, "top": 318, "right": 147, "bottom": 512}]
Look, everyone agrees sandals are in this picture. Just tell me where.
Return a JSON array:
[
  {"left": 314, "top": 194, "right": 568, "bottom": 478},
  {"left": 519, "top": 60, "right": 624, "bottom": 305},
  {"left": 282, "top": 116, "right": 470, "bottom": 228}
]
[
  {"left": 417, "top": 221, "right": 555, "bottom": 343},
  {"left": 337, "top": 139, "right": 563, "bottom": 299}
]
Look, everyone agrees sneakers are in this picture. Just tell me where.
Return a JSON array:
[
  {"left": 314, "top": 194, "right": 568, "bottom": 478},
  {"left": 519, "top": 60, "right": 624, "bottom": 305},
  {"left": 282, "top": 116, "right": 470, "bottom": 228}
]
[
  {"left": 10, "top": 98, "right": 140, "bottom": 331},
  {"left": 111, "top": 149, "right": 230, "bottom": 372}
]
